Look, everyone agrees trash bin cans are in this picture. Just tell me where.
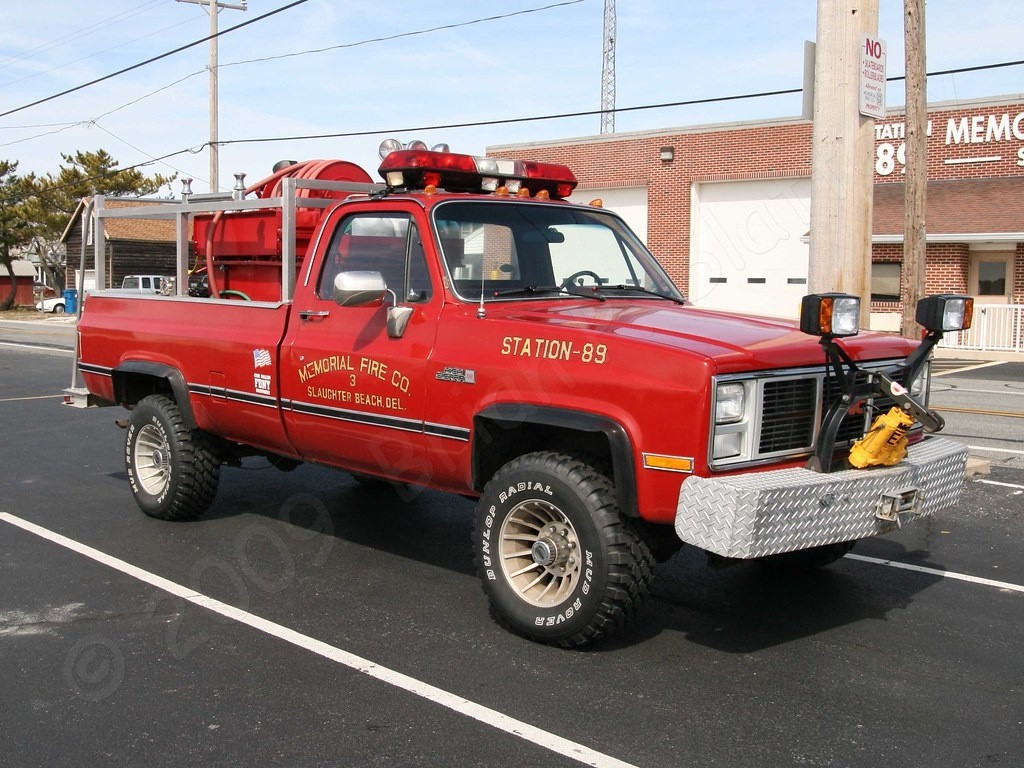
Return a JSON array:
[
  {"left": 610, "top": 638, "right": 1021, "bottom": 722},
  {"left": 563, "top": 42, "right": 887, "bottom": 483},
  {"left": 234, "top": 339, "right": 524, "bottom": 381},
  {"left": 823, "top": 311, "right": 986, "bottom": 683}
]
[{"left": 61, "top": 288, "right": 78, "bottom": 314}]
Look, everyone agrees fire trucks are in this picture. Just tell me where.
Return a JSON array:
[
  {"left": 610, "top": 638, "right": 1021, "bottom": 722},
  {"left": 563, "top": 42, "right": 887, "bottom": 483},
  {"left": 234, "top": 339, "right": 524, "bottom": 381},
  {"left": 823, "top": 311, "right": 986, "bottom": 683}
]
[{"left": 60, "top": 140, "right": 975, "bottom": 653}]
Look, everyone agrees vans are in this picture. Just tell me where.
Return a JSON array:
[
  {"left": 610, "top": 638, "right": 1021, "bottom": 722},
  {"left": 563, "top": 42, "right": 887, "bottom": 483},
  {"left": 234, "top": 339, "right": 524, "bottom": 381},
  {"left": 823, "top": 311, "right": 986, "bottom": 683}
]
[{"left": 122, "top": 275, "right": 164, "bottom": 296}]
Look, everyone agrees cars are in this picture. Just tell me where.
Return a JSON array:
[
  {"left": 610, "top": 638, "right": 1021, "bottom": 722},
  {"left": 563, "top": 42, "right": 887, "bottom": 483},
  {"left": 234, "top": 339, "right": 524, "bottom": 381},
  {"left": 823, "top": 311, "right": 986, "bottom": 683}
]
[{"left": 36, "top": 297, "right": 65, "bottom": 314}]
